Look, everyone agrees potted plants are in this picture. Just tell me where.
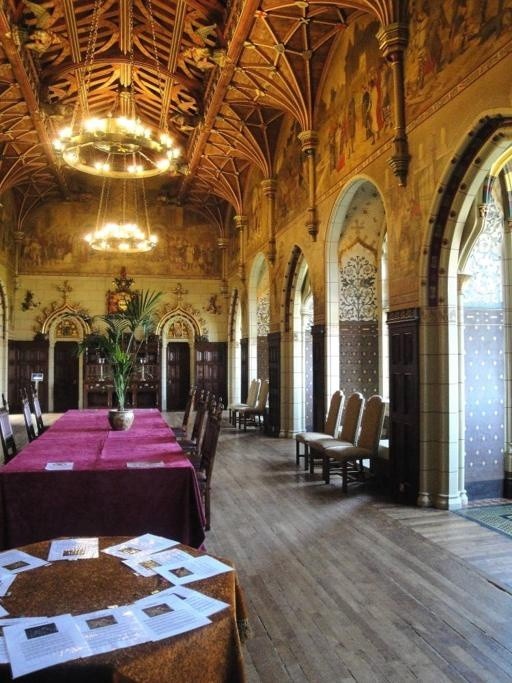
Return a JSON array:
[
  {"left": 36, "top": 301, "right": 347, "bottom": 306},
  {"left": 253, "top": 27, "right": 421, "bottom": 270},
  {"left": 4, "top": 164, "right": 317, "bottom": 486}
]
[{"left": 75, "top": 288, "right": 163, "bottom": 431}]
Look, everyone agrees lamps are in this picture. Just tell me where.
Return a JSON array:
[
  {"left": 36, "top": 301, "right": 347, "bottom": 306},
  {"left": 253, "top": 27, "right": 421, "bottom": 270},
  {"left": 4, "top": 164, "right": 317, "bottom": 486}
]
[{"left": 53, "top": 0, "right": 182, "bottom": 252}]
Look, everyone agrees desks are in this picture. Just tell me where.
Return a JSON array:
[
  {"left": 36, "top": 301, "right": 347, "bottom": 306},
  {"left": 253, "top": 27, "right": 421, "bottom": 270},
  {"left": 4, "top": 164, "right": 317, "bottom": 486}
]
[
  {"left": 1, "top": 411, "right": 208, "bottom": 544},
  {"left": 0, "top": 539, "right": 254, "bottom": 683}
]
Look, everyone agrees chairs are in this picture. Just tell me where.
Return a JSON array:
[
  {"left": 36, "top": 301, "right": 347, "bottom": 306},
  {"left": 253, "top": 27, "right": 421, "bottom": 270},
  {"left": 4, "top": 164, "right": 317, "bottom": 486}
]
[
  {"left": 0, "top": 383, "right": 46, "bottom": 466},
  {"left": 228, "top": 379, "right": 270, "bottom": 433},
  {"left": 171, "top": 384, "right": 224, "bottom": 530},
  {"left": 295, "top": 391, "right": 386, "bottom": 494}
]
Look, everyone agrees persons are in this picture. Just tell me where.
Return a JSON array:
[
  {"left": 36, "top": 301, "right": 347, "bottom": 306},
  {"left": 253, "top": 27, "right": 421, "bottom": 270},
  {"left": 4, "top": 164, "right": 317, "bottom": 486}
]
[
  {"left": 20, "top": 227, "right": 220, "bottom": 276},
  {"left": 326, "top": 0, "right": 510, "bottom": 173}
]
